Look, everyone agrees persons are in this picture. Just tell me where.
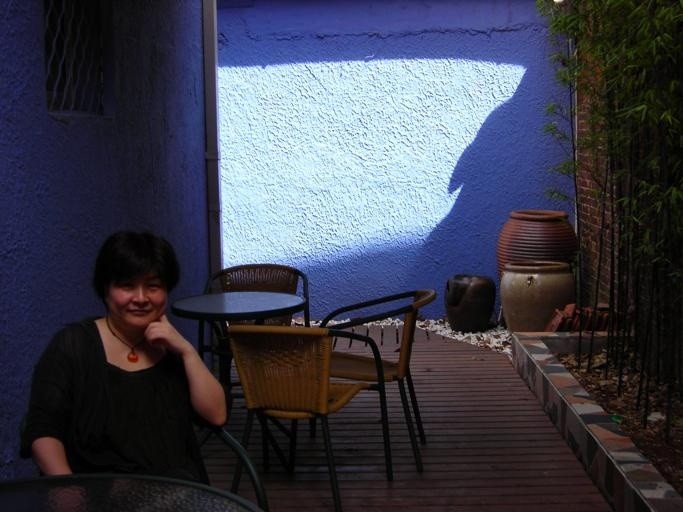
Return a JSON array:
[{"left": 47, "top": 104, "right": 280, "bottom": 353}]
[{"left": 17, "top": 228, "right": 229, "bottom": 510}]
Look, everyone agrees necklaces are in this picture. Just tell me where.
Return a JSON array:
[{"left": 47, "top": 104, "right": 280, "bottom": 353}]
[{"left": 103, "top": 314, "right": 146, "bottom": 362}]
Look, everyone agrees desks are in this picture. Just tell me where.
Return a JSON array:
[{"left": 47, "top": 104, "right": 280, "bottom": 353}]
[{"left": 171, "top": 292, "right": 307, "bottom": 473}]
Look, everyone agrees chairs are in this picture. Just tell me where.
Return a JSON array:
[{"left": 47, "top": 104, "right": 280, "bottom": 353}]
[
  {"left": 226, "top": 326, "right": 393, "bottom": 512},
  {"left": 0, "top": 474, "right": 266, "bottom": 511},
  {"left": 21, "top": 425, "right": 269, "bottom": 511},
  {"left": 198, "top": 264, "right": 310, "bottom": 361},
  {"left": 309, "top": 289, "right": 436, "bottom": 463}
]
